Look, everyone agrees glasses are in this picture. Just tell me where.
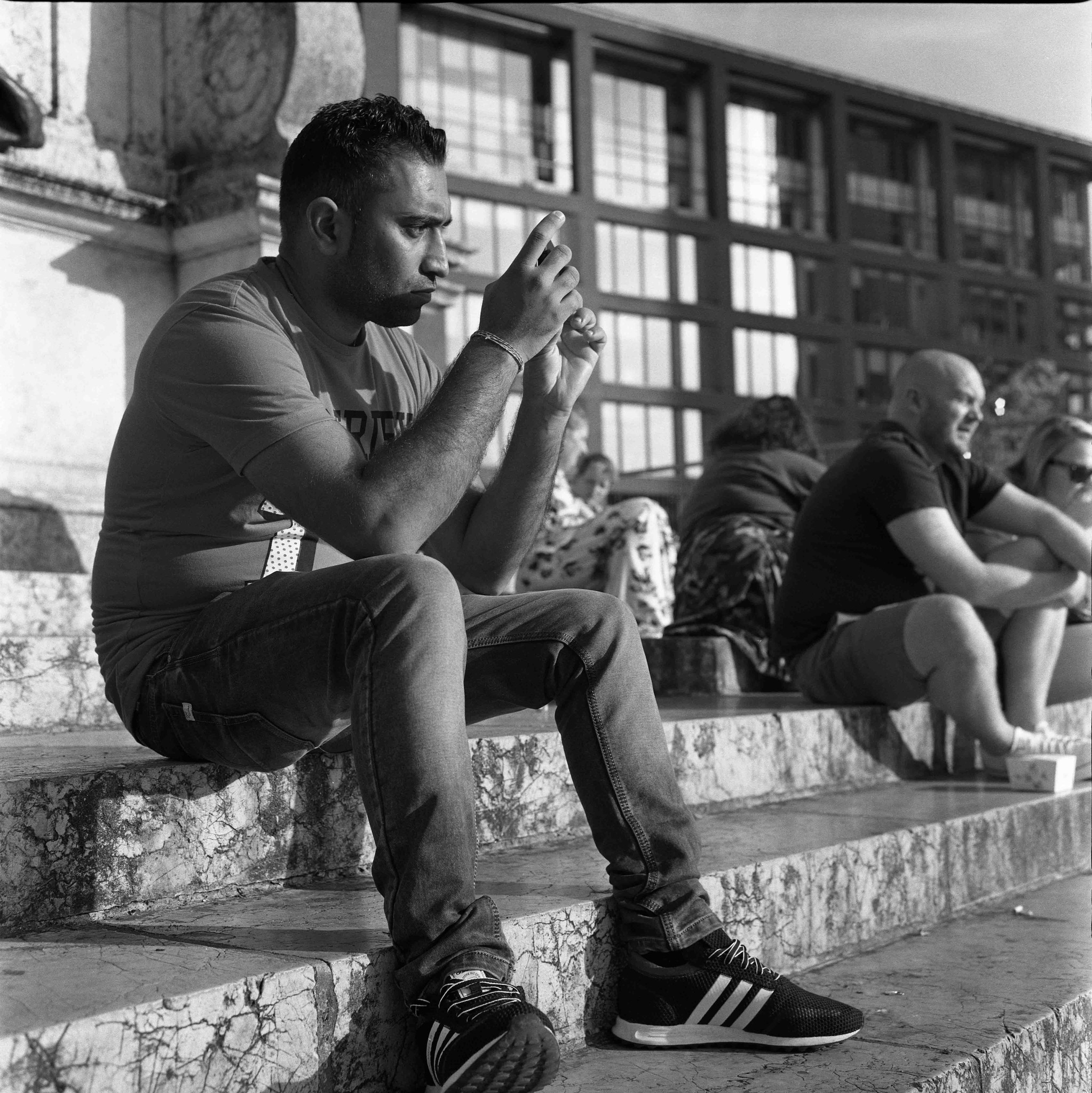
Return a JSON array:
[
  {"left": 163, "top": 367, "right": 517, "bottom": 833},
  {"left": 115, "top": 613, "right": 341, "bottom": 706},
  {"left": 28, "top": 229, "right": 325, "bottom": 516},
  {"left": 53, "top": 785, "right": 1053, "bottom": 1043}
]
[{"left": 1052, "top": 459, "right": 1092, "bottom": 483}]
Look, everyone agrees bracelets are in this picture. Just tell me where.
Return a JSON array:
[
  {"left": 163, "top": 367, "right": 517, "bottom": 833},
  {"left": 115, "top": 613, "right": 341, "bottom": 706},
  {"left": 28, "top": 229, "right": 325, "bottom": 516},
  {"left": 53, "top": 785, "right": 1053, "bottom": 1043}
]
[{"left": 469, "top": 329, "right": 523, "bottom": 373}]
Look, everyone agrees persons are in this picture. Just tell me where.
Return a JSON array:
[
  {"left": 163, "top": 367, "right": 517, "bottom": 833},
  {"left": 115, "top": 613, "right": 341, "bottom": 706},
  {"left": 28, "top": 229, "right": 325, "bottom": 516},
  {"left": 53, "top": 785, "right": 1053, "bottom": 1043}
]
[
  {"left": 502, "top": 351, "right": 1092, "bottom": 786},
  {"left": 92, "top": 93, "right": 865, "bottom": 1093}
]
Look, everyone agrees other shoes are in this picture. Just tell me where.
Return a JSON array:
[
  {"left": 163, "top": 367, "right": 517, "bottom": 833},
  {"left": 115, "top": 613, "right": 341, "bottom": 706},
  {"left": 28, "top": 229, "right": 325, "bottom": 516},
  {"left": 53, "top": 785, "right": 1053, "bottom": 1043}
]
[
  {"left": 422, "top": 968, "right": 560, "bottom": 1093},
  {"left": 612, "top": 929, "right": 863, "bottom": 1048},
  {"left": 981, "top": 719, "right": 1092, "bottom": 785}
]
